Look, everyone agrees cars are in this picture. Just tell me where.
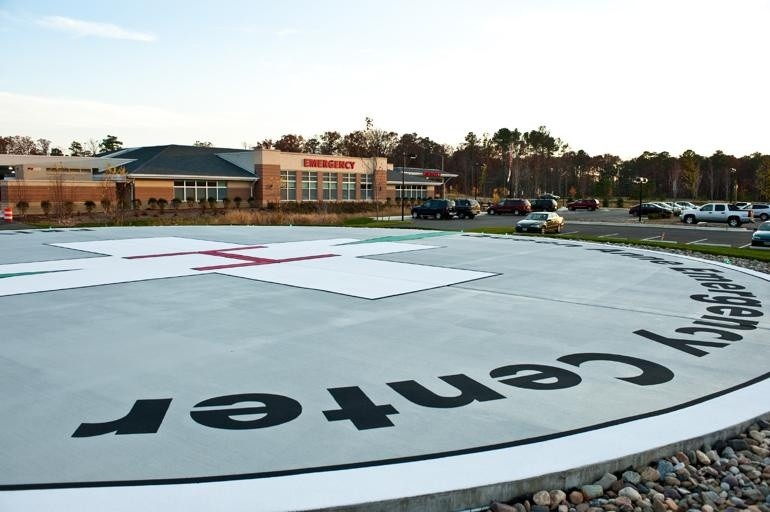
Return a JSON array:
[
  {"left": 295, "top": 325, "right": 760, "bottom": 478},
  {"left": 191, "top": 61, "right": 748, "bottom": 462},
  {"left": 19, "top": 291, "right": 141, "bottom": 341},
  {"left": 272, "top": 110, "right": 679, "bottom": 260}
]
[
  {"left": 629, "top": 201, "right": 770, "bottom": 246},
  {"left": 515, "top": 212, "right": 565, "bottom": 234}
]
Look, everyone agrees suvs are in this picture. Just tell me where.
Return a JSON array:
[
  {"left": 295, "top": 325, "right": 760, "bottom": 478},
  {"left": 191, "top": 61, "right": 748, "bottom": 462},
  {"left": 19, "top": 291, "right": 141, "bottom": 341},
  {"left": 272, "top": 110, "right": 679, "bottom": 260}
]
[{"left": 487, "top": 193, "right": 599, "bottom": 214}]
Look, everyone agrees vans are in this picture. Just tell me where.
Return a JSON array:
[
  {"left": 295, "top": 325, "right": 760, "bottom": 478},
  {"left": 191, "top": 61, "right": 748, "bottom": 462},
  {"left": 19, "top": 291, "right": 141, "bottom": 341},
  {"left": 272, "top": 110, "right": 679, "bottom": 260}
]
[{"left": 411, "top": 198, "right": 481, "bottom": 220}]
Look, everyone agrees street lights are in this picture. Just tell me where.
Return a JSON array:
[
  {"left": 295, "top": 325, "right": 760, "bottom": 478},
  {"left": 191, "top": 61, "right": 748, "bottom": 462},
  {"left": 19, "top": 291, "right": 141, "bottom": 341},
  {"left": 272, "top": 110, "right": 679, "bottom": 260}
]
[
  {"left": 633, "top": 176, "right": 648, "bottom": 221},
  {"left": 475, "top": 162, "right": 485, "bottom": 199},
  {"left": 401, "top": 151, "right": 418, "bottom": 221},
  {"left": 439, "top": 153, "right": 449, "bottom": 199},
  {"left": 729, "top": 167, "right": 736, "bottom": 203}
]
